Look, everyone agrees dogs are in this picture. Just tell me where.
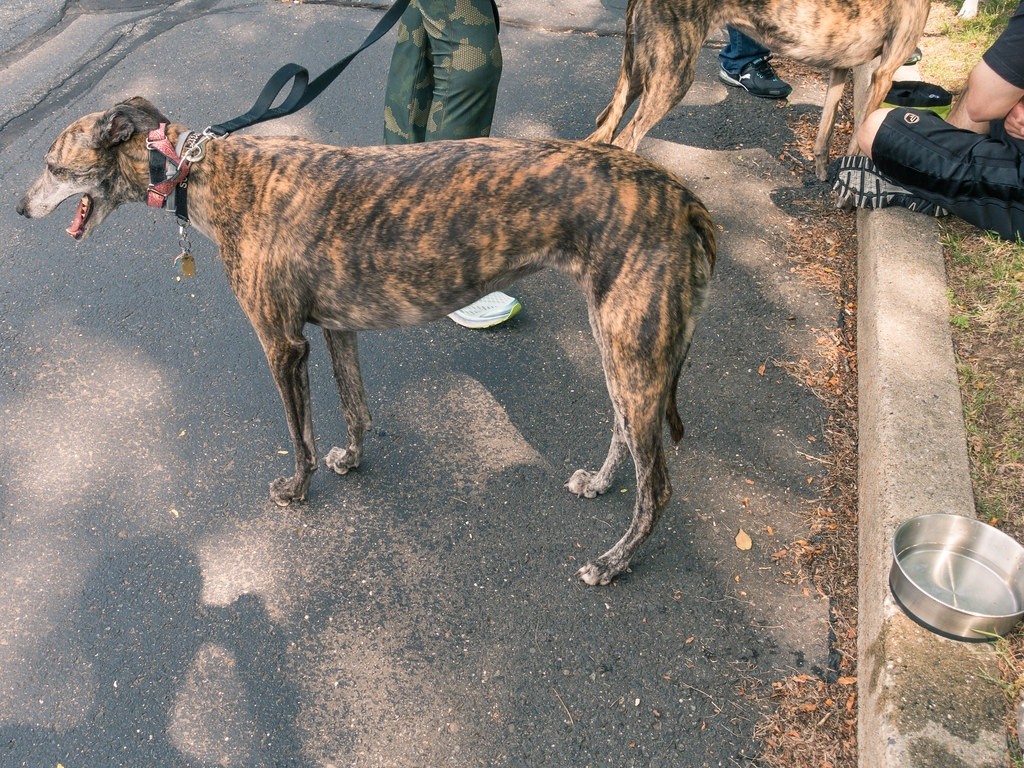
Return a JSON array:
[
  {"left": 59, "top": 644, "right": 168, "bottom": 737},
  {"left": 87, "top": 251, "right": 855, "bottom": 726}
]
[
  {"left": 14, "top": 96, "right": 719, "bottom": 587},
  {"left": 584, "top": 0, "right": 932, "bottom": 183}
]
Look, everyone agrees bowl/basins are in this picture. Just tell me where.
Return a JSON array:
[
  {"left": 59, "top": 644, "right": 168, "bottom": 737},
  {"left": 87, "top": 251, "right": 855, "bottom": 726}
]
[{"left": 890, "top": 515, "right": 1024, "bottom": 642}]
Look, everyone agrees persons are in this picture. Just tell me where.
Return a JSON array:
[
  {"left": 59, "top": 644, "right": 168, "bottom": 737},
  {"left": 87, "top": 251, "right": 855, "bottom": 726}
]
[
  {"left": 825, "top": 0, "right": 1024, "bottom": 246},
  {"left": 718, "top": 23, "right": 921, "bottom": 98},
  {"left": 381, "top": 0, "right": 522, "bottom": 329}
]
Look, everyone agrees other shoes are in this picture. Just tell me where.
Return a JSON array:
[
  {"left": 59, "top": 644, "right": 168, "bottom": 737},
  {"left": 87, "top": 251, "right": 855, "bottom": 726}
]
[{"left": 826, "top": 156, "right": 950, "bottom": 218}]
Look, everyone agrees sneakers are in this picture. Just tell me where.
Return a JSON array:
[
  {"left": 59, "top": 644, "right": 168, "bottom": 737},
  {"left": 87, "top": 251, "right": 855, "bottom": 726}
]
[
  {"left": 446, "top": 291, "right": 522, "bottom": 329},
  {"left": 719, "top": 55, "right": 793, "bottom": 99},
  {"left": 901, "top": 47, "right": 922, "bottom": 66}
]
